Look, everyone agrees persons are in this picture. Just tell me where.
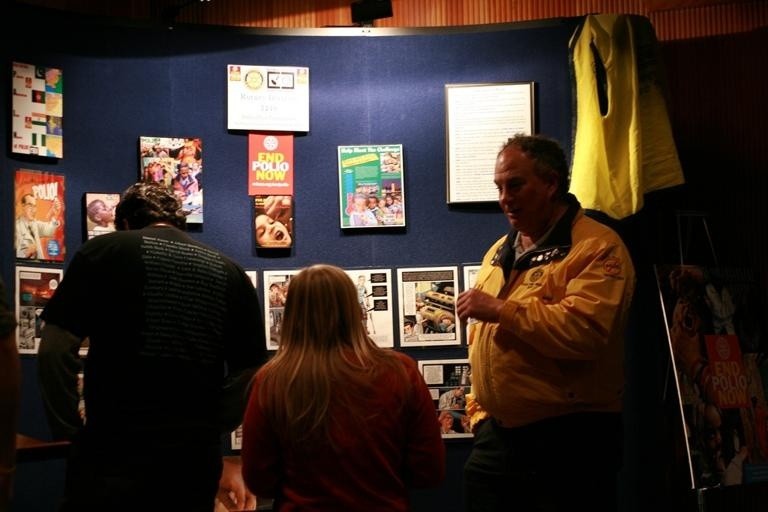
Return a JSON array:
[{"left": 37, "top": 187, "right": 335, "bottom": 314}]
[
  {"left": 436, "top": 386, "right": 466, "bottom": 410},
  {"left": 254, "top": 210, "right": 293, "bottom": 246},
  {"left": 347, "top": 191, "right": 379, "bottom": 225},
  {"left": 238, "top": 261, "right": 447, "bottom": 512},
  {"left": 454, "top": 130, "right": 635, "bottom": 512},
  {"left": 365, "top": 191, "right": 401, "bottom": 223},
  {"left": 437, "top": 413, "right": 458, "bottom": 435},
  {"left": 87, "top": 199, "right": 115, "bottom": 240},
  {"left": 268, "top": 284, "right": 285, "bottom": 305},
  {"left": 0, "top": 272, "right": 24, "bottom": 512},
  {"left": 14, "top": 192, "right": 62, "bottom": 260},
  {"left": 36, "top": 182, "right": 266, "bottom": 512},
  {"left": 170, "top": 162, "right": 199, "bottom": 214}
]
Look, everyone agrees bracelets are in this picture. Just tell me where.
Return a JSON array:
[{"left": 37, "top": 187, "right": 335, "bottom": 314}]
[{"left": 1, "top": 465, "right": 20, "bottom": 476}]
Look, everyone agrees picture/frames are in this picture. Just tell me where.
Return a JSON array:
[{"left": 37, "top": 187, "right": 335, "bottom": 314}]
[
  {"left": 80, "top": 189, "right": 123, "bottom": 245},
  {"left": 229, "top": 423, "right": 242, "bottom": 451},
  {"left": 261, "top": 266, "right": 307, "bottom": 354},
  {"left": 415, "top": 357, "right": 480, "bottom": 440},
  {"left": 338, "top": 264, "right": 397, "bottom": 350},
  {"left": 242, "top": 265, "right": 259, "bottom": 292},
  {"left": 9, "top": 258, "right": 67, "bottom": 361},
  {"left": 394, "top": 261, "right": 461, "bottom": 355},
  {"left": 13, "top": 165, "right": 69, "bottom": 266},
  {"left": 442, "top": 77, "right": 538, "bottom": 208},
  {"left": 461, "top": 259, "right": 487, "bottom": 349}
]
